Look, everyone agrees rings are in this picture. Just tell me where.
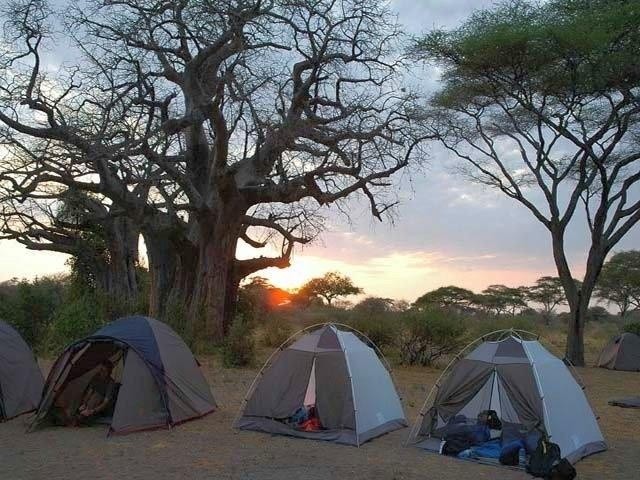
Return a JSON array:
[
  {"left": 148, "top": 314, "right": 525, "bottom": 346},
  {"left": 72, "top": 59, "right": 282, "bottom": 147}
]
[{"left": 87, "top": 414, "right": 88, "bottom": 416}]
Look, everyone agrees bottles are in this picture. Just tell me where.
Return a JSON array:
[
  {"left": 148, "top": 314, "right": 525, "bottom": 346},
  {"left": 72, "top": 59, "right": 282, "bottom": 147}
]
[{"left": 518, "top": 448, "right": 527, "bottom": 469}]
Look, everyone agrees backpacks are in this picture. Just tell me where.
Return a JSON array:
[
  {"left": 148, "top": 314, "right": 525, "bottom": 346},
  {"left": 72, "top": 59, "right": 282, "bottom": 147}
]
[{"left": 523, "top": 436, "right": 579, "bottom": 480}]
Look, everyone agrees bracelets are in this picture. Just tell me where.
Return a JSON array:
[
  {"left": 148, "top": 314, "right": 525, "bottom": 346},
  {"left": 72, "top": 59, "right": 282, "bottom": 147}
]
[{"left": 93, "top": 409, "right": 98, "bottom": 417}]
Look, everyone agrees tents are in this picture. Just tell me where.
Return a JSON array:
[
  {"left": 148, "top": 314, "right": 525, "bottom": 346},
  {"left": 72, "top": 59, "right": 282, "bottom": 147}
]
[
  {"left": 596, "top": 332, "right": 640, "bottom": 372},
  {"left": 0, "top": 318, "right": 66, "bottom": 423},
  {"left": 231, "top": 321, "right": 410, "bottom": 448},
  {"left": 25, "top": 313, "right": 219, "bottom": 439},
  {"left": 403, "top": 326, "right": 609, "bottom": 474}
]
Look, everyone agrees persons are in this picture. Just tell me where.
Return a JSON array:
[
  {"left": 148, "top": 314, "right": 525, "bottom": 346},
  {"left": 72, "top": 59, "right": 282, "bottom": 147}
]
[{"left": 74, "top": 359, "right": 116, "bottom": 428}]
